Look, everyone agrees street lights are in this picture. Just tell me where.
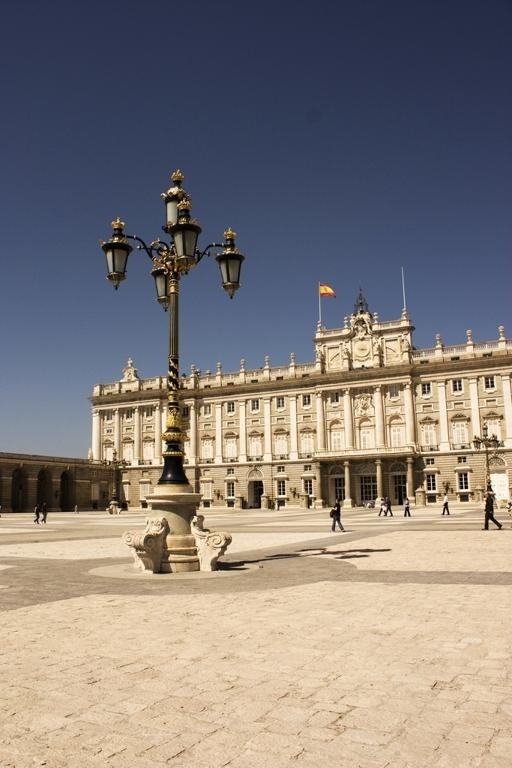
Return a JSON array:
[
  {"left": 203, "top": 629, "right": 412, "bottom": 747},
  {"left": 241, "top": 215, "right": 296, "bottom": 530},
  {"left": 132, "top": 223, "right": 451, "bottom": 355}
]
[
  {"left": 100, "top": 448, "right": 132, "bottom": 501},
  {"left": 93, "top": 165, "right": 250, "bottom": 484},
  {"left": 470, "top": 423, "right": 505, "bottom": 493}
]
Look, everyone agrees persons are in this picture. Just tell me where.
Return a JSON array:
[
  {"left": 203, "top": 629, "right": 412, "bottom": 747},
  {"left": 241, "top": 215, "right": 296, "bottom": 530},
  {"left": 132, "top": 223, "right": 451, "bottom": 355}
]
[
  {"left": 481, "top": 492, "right": 502, "bottom": 530},
  {"left": 383, "top": 496, "right": 393, "bottom": 516},
  {"left": 329, "top": 497, "right": 344, "bottom": 532},
  {"left": 33, "top": 503, "right": 41, "bottom": 524},
  {"left": 41, "top": 501, "right": 48, "bottom": 523},
  {"left": 75, "top": 504, "right": 80, "bottom": 514},
  {"left": 377, "top": 498, "right": 387, "bottom": 516},
  {"left": 403, "top": 497, "right": 411, "bottom": 517},
  {"left": 440, "top": 492, "right": 449, "bottom": 515}
]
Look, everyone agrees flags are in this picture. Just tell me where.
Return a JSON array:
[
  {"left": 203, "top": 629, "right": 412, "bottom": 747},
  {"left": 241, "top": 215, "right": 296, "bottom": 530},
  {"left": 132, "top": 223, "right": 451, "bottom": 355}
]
[{"left": 319, "top": 282, "right": 337, "bottom": 298}]
